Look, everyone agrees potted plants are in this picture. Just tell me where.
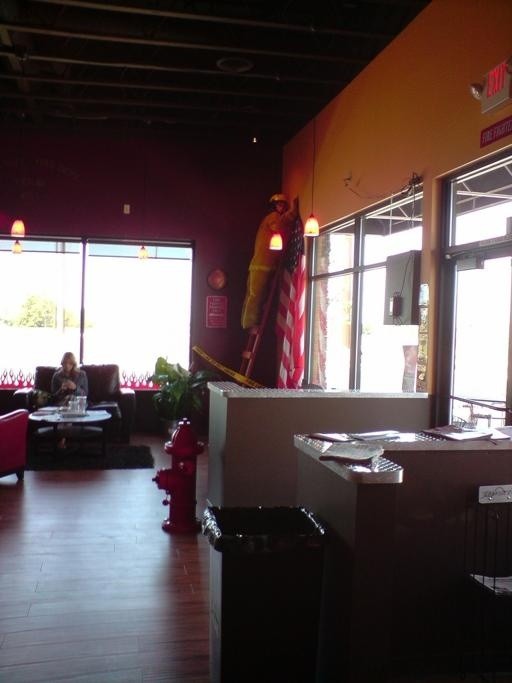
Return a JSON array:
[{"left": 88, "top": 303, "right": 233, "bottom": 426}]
[{"left": 148, "top": 357, "right": 223, "bottom": 435}]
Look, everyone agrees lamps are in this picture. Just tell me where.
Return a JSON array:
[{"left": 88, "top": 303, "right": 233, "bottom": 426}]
[
  {"left": 269, "top": 25, "right": 320, "bottom": 251},
  {"left": 10, "top": 46, "right": 26, "bottom": 256},
  {"left": 470, "top": 56, "right": 512, "bottom": 115},
  {"left": 137, "top": 121, "right": 151, "bottom": 259}
]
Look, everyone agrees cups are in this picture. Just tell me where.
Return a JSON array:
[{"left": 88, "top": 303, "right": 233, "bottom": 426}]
[
  {"left": 68, "top": 400, "right": 78, "bottom": 413},
  {"left": 76, "top": 396, "right": 87, "bottom": 414}
]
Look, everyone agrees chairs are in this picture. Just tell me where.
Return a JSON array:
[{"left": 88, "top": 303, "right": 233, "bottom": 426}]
[
  {"left": 0, "top": 408, "right": 29, "bottom": 481},
  {"left": 15, "top": 365, "right": 136, "bottom": 445}
]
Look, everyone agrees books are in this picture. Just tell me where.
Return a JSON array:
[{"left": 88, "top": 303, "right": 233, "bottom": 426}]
[
  {"left": 320, "top": 441, "right": 385, "bottom": 466},
  {"left": 310, "top": 432, "right": 348, "bottom": 443},
  {"left": 352, "top": 428, "right": 403, "bottom": 441},
  {"left": 474, "top": 428, "right": 510, "bottom": 440},
  {"left": 427, "top": 423, "right": 494, "bottom": 443}
]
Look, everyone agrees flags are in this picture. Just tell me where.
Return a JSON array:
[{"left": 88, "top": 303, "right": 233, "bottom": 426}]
[{"left": 264, "top": 212, "right": 306, "bottom": 389}]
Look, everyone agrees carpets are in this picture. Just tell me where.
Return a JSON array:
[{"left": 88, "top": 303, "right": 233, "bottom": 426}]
[{"left": 26, "top": 445, "right": 155, "bottom": 471}]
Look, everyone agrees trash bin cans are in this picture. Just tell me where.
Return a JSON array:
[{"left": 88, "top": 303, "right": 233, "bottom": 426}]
[{"left": 201, "top": 505, "right": 331, "bottom": 683}]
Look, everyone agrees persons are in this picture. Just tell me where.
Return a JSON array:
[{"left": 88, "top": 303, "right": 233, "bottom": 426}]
[
  {"left": 240, "top": 192, "right": 298, "bottom": 330},
  {"left": 51, "top": 352, "right": 89, "bottom": 450}
]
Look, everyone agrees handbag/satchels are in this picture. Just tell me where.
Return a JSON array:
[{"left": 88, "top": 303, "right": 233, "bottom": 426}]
[{"left": 32, "top": 389, "right": 49, "bottom": 406}]
